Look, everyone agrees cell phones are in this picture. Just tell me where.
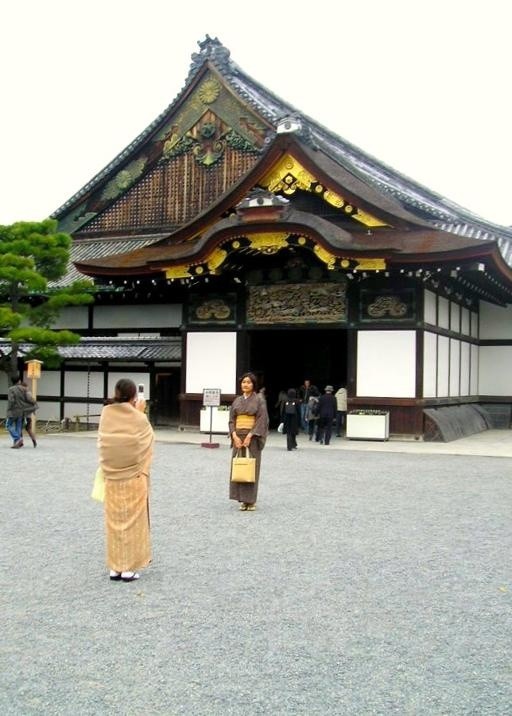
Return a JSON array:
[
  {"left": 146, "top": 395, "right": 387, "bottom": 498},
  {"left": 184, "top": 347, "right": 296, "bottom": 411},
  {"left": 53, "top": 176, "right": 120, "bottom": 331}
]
[{"left": 138, "top": 384, "right": 144, "bottom": 402}]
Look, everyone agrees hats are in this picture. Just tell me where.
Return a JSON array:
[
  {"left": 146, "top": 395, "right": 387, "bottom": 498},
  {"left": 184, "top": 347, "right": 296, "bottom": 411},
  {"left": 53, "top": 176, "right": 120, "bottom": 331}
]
[{"left": 324, "top": 385, "right": 334, "bottom": 391}]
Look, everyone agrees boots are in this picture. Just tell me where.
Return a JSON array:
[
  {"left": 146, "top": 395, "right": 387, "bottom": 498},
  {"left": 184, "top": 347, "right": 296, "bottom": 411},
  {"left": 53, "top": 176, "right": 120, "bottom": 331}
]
[{"left": 32, "top": 440, "right": 37, "bottom": 449}]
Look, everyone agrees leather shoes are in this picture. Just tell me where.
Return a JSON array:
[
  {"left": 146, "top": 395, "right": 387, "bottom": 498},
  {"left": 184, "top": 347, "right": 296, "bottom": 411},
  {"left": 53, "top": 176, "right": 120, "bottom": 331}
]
[
  {"left": 239, "top": 501, "right": 247, "bottom": 511},
  {"left": 247, "top": 504, "right": 255, "bottom": 511}
]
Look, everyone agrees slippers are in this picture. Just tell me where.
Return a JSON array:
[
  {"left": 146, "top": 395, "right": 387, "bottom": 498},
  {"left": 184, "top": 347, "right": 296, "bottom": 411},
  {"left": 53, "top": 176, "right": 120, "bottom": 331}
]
[
  {"left": 110, "top": 573, "right": 121, "bottom": 579},
  {"left": 122, "top": 573, "right": 139, "bottom": 581}
]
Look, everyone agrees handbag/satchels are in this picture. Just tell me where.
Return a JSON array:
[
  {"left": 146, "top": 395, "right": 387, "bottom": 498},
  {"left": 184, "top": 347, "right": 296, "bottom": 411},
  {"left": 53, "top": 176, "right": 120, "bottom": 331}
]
[{"left": 230, "top": 445, "right": 256, "bottom": 483}]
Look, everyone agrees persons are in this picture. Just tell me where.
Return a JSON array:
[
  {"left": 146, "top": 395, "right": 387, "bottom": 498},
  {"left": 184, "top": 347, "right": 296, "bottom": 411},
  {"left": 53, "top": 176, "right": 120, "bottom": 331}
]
[
  {"left": 228, "top": 371, "right": 268, "bottom": 511},
  {"left": 20, "top": 383, "right": 37, "bottom": 449},
  {"left": 274, "top": 380, "right": 348, "bottom": 451},
  {"left": 5, "top": 374, "right": 40, "bottom": 448},
  {"left": 91, "top": 378, "right": 156, "bottom": 582}
]
